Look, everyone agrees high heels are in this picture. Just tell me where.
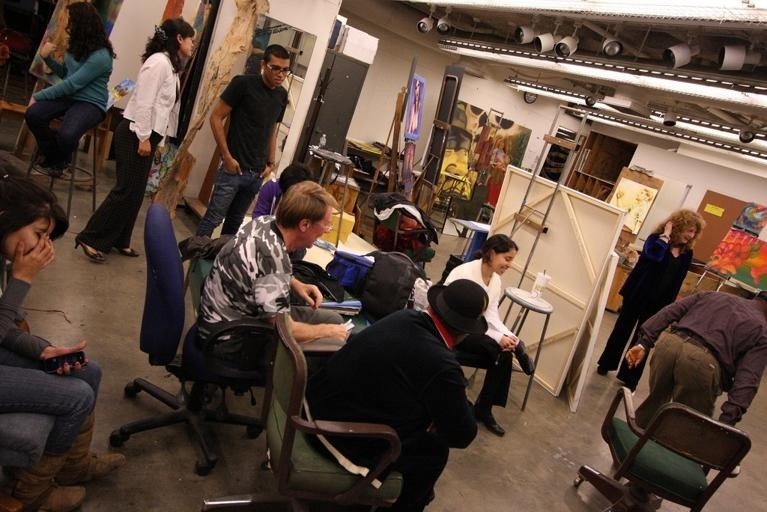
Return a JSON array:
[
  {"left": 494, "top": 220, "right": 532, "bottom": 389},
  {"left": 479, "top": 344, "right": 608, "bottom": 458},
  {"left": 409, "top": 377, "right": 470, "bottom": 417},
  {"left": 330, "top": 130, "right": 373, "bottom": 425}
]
[
  {"left": 119, "top": 248, "right": 139, "bottom": 257},
  {"left": 74, "top": 237, "right": 105, "bottom": 261}
]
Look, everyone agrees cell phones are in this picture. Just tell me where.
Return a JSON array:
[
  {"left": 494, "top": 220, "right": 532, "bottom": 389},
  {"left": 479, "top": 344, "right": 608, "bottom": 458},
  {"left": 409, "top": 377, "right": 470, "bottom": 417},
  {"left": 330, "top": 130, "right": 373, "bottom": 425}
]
[{"left": 45, "top": 351, "right": 88, "bottom": 373}]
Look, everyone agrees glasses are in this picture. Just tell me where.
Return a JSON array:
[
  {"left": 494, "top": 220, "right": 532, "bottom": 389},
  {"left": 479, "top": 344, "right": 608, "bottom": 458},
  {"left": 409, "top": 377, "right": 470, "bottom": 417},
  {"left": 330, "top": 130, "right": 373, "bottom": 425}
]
[
  {"left": 312, "top": 221, "right": 334, "bottom": 233},
  {"left": 264, "top": 64, "right": 291, "bottom": 77}
]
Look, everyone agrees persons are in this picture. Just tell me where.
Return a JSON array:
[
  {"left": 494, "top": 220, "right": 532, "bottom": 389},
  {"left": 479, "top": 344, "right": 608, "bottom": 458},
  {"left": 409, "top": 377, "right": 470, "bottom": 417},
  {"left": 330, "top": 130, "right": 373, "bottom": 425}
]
[
  {"left": 23, "top": 1, "right": 118, "bottom": 179},
  {"left": 2, "top": 174, "right": 125, "bottom": 510},
  {"left": 75, "top": 19, "right": 194, "bottom": 260},
  {"left": 179, "top": 165, "right": 535, "bottom": 510},
  {"left": 194, "top": 44, "right": 290, "bottom": 238},
  {"left": 596, "top": 208, "right": 707, "bottom": 393},
  {"left": 623, "top": 291, "right": 766, "bottom": 435}
]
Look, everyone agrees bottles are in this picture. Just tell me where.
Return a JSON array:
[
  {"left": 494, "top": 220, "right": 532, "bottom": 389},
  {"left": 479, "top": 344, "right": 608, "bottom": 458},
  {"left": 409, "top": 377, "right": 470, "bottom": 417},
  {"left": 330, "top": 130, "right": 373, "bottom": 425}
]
[{"left": 318, "top": 133, "right": 326, "bottom": 151}]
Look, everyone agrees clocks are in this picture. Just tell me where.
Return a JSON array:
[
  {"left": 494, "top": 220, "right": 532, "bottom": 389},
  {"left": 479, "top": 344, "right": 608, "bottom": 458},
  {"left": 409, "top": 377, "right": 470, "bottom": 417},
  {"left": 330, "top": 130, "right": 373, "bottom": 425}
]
[{"left": 523, "top": 90, "right": 539, "bottom": 103}]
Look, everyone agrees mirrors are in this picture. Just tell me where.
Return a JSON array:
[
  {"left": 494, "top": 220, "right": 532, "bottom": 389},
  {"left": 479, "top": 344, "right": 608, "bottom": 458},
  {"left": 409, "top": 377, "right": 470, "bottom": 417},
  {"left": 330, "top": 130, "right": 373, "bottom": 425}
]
[{"left": 195, "top": 13, "right": 318, "bottom": 214}]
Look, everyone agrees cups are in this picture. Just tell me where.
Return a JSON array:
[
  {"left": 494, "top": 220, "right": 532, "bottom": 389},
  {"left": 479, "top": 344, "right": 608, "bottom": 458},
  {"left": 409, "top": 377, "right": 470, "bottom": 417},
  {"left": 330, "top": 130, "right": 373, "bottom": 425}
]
[{"left": 529, "top": 271, "right": 551, "bottom": 302}]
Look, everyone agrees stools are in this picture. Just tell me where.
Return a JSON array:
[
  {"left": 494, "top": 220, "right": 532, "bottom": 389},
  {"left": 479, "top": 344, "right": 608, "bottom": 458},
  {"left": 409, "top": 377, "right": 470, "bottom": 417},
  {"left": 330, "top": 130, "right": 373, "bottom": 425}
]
[
  {"left": 25, "top": 116, "right": 97, "bottom": 222},
  {"left": 309, "top": 143, "right": 354, "bottom": 251},
  {"left": 497, "top": 286, "right": 555, "bottom": 413}
]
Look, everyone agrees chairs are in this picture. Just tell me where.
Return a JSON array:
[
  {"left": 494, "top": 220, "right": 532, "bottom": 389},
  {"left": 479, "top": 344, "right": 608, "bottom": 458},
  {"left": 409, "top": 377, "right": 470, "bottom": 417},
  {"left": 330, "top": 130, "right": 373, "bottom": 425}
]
[
  {"left": 203, "top": 312, "right": 403, "bottom": 511},
  {"left": 107, "top": 201, "right": 265, "bottom": 476},
  {"left": 371, "top": 202, "right": 435, "bottom": 273},
  {"left": 574, "top": 388, "right": 754, "bottom": 511}
]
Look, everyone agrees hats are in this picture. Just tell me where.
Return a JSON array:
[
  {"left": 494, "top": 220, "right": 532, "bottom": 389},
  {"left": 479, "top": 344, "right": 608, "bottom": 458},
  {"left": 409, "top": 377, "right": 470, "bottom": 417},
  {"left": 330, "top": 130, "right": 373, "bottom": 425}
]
[{"left": 427, "top": 279, "right": 488, "bottom": 335}]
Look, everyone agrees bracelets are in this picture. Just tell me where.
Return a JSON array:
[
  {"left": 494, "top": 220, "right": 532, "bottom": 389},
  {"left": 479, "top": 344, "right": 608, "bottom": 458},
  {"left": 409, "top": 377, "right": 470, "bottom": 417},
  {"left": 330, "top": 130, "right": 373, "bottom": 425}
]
[
  {"left": 658, "top": 233, "right": 670, "bottom": 241},
  {"left": 267, "top": 159, "right": 276, "bottom": 167},
  {"left": 638, "top": 343, "right": 645, "bottom": 351}
]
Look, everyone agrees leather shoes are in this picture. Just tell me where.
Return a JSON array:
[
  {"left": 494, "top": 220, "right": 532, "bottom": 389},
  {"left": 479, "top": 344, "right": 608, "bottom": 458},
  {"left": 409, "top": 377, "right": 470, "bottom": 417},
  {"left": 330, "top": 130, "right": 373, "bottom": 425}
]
[
  {"left": 473, "top": 404, "right": 504, "bottom": 435},
  {"left": 513, "top": 339, "right": 534, "bottom": 375}
]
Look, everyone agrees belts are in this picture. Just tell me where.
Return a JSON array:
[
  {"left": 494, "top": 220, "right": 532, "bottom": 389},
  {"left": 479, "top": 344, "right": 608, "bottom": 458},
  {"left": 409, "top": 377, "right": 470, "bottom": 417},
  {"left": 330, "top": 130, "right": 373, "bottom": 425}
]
[{"left": 673, "top": 330, "right": 705, "bottom": 353}]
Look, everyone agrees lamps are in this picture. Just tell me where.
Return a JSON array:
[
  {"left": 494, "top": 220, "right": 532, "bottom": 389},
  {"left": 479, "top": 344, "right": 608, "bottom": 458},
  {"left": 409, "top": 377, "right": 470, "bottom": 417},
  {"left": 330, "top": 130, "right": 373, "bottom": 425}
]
[
  {"left": 414, "top": 6, "right": 767, "bottom": 72},
  {"left": 583, "top": 92, "right": 755, "bottom": 143}
]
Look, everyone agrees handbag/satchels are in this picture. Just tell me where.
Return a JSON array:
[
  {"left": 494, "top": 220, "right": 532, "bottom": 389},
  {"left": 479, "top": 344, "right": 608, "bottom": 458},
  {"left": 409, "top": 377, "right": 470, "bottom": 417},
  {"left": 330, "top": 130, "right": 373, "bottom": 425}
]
[
  {"left": 324, "top": 250, "right": 374, "bottom": 297},
  {"left": 359, "top": 251, "right": 430, "bottom": 324}
]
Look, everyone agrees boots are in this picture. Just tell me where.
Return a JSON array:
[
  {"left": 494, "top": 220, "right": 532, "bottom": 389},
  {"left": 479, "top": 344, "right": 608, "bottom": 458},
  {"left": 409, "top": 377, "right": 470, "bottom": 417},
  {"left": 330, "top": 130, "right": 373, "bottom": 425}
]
[
  {"left": 10, "top": 453, "right": 86, "bottom": 511},
  {"left": 54, "top": 425, "right": 125, "bottom": 486}
]
